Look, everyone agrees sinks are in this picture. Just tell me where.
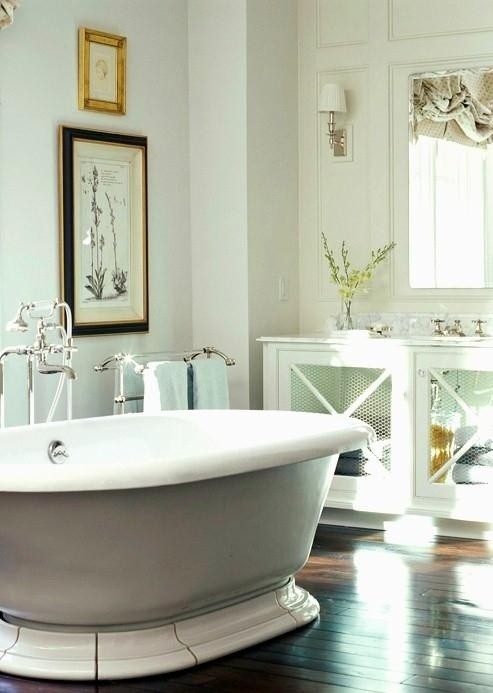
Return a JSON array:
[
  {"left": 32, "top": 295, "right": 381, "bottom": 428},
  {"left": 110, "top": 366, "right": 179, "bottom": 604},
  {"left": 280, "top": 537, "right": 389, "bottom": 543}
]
[{"left": 409, "top": 337, "right": 479, "bottom": 344}]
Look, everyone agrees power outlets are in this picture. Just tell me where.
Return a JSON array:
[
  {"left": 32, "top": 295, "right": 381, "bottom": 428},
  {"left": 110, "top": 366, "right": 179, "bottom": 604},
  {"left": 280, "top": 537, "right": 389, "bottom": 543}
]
[{"left": 278, "top": 277, "right": 292, "bottom": 303}]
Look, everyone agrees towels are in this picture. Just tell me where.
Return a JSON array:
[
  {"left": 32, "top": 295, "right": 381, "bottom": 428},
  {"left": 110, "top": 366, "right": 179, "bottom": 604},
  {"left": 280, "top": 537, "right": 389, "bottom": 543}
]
[
  {"left": 189, "top": 358, "right": 228, "bottom": 410},
  {"left": 144, "top": 362, "right": 189, "bottom": 412}
]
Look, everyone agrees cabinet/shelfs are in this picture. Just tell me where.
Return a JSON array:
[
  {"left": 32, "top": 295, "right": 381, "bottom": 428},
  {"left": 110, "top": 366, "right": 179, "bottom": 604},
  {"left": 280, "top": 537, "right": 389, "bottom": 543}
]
[{"left": 255, "top": 330, "right": 493, "bottom": 541}]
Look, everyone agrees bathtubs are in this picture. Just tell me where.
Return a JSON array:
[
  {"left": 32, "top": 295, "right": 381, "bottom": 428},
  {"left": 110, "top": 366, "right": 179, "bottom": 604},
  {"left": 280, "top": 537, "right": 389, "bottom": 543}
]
[{"left": 0, "top": 410, "right": 374, "bottom": 681}]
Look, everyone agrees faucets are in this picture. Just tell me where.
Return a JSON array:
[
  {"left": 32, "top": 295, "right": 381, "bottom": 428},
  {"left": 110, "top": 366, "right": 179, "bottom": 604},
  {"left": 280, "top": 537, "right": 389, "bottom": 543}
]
[
  {"left": 444, "top": 319, "right": 466, "bottom": 336},
  {"left": 0, "top": 340, "right": 78, "bottom": 424}
]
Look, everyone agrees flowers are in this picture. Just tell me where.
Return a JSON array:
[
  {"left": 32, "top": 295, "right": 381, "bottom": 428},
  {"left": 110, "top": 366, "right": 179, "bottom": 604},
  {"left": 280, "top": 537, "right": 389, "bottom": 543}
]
[{"left": 321, "top": 231, "right": 399, "bottom": 332}]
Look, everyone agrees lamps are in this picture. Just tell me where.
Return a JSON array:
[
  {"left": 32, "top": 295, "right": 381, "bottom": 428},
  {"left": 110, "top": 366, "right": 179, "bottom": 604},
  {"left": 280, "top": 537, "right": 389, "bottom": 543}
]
[{"left": 316, "top": 81, "right": 347, "bottom": 158}]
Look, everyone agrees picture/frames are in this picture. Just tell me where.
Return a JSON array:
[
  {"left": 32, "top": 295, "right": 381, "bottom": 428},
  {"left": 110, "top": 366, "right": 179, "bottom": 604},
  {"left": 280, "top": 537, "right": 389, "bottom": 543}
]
[
  {"left": 56, "top": 123, "right": 150, "bottom": 336},
  {"left": 77, "top": 26, "right": 126, "bottom": 115}
]
